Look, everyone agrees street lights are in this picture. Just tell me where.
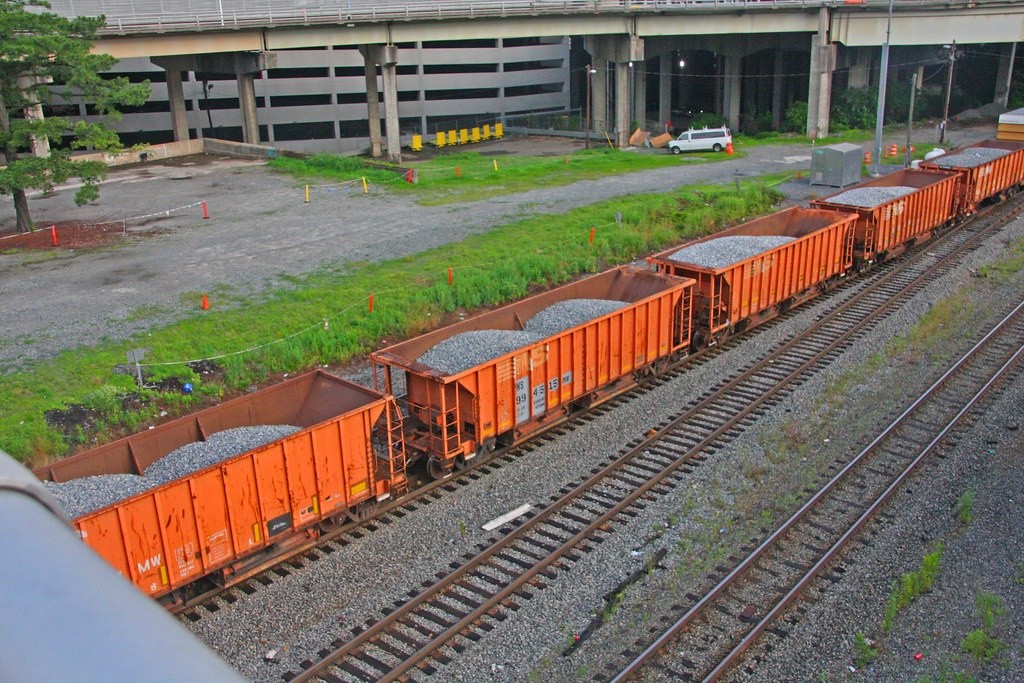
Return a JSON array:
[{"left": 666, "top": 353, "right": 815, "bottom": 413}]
[
  {"left": 586, "top": 64, "right": 597, "bottom": 149},
  {"left": 203, "top": 79, "right": 214, "bottom": 129},
  {"left": 941, "top": 40, "right": 955, "bottom": 142}
]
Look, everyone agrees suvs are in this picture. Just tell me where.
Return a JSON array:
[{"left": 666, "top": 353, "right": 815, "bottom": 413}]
[{"left": 667, "top": 126, "right": 732, "bottom": 154}]
